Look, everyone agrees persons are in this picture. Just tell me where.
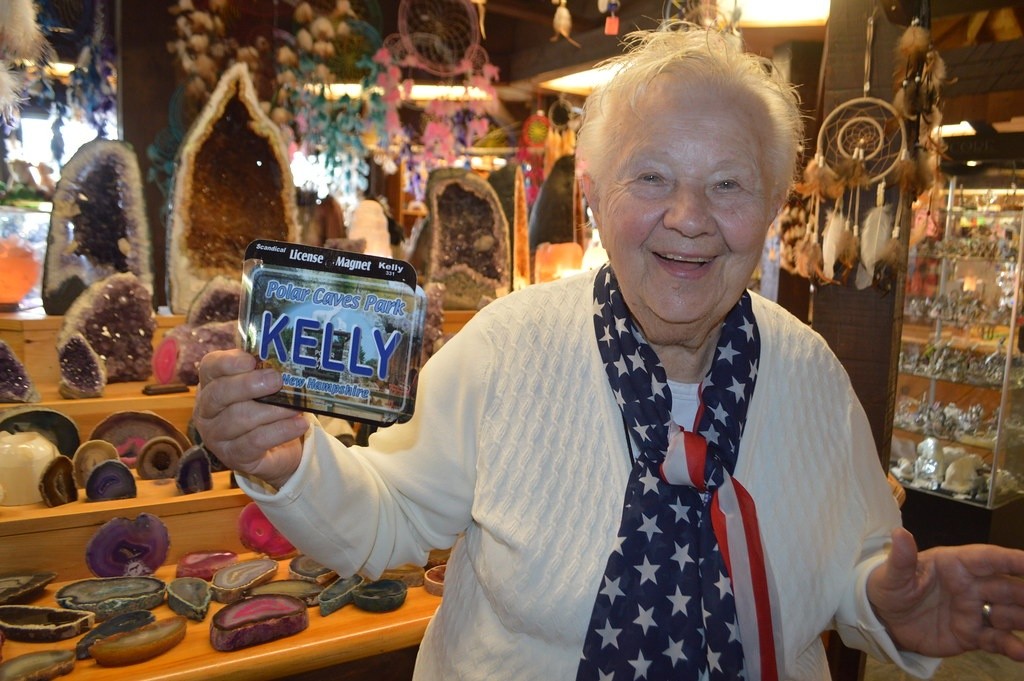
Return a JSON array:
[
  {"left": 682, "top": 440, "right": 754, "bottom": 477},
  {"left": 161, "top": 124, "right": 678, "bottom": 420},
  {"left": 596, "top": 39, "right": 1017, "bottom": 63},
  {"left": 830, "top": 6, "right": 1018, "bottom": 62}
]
[{"left": 194, "top": 20, "right": 1024, "bottom": 681}]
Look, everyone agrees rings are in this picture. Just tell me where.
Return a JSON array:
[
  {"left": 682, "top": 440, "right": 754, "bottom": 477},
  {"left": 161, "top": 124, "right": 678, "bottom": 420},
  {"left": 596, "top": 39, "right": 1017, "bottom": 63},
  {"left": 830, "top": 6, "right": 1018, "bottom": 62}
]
[{"left": 981, "top": 602, "right": 994, "bottom": 628}]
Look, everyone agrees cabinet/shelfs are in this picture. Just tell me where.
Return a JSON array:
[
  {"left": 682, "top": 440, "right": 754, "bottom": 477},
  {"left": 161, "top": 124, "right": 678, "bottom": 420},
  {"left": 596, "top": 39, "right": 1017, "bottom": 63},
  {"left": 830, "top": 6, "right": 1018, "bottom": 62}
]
[{"left": 890, "top": 189, "right": 1024, "bottom": 510}]
